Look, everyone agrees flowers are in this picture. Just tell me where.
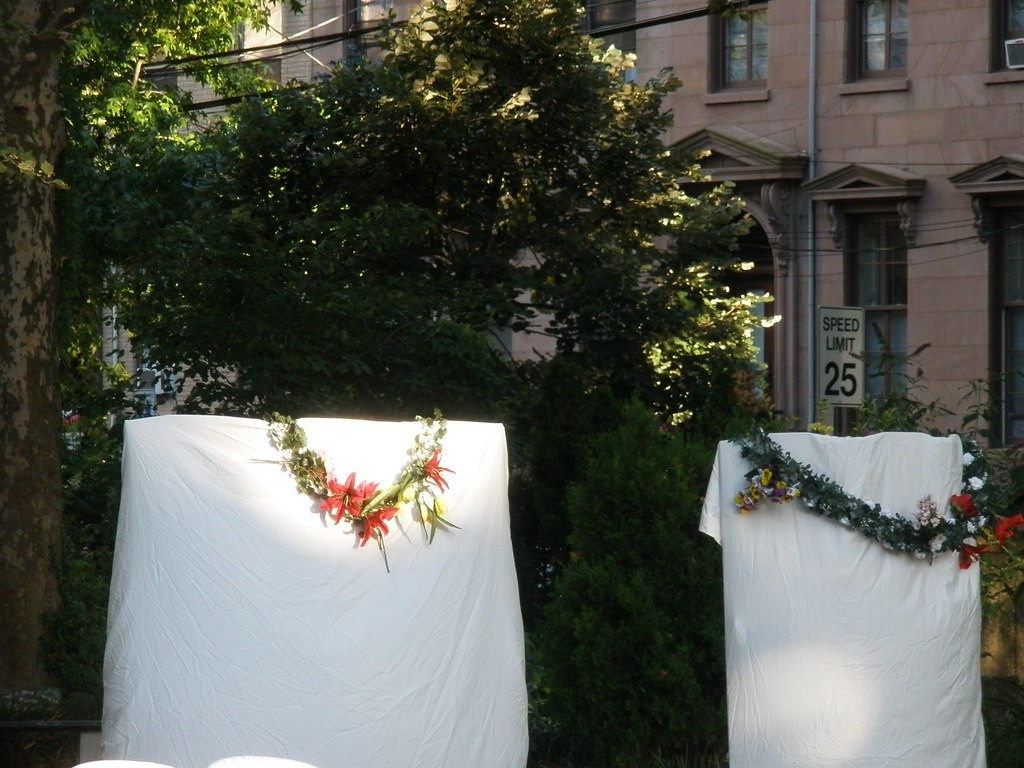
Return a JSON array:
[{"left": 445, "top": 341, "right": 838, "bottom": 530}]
[
  {"left": 265, "top": 405, "right": 464, "bottom": 569},
  {"left": 947, "top": 494, "right": 1023, "bottom": 569}
]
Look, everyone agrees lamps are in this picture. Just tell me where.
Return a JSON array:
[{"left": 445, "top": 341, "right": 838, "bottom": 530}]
[{"left": 1004, "top": 38, "right": 1024, "bottom": 68}]
